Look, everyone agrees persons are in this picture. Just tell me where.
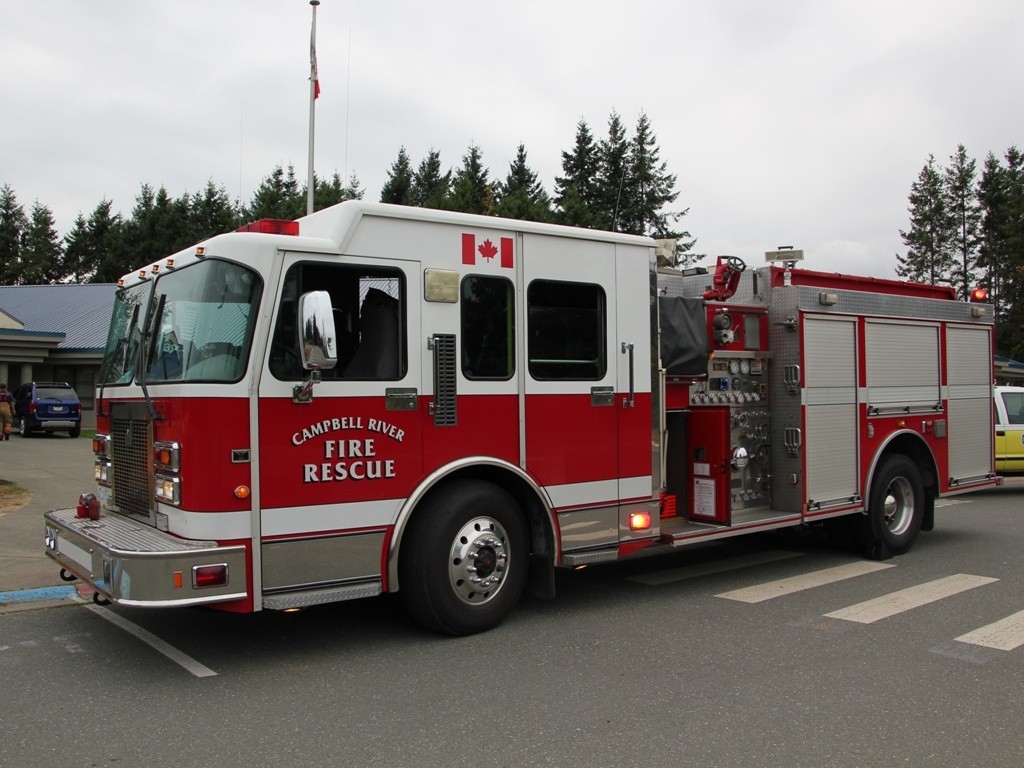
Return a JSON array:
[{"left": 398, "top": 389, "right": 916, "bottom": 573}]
[
  {"left": 304, "top": 340, "right": 325, "bottom": 368},
  {"left": 0, "top": 383, "right": 17, "bottom": 441}
]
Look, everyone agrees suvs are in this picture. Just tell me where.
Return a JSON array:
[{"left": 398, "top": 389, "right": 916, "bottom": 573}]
[{"left": 13, "top": 380, "right": 83, "bottom": 438}]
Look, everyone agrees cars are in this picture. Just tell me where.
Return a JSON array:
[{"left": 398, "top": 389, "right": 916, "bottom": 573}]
[{"left": 992, "top": 384, "right": 1024, "bottom": 474}]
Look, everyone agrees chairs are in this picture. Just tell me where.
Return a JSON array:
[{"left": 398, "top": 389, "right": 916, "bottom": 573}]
[{"left": 344, "top": 286, "right": 399, "bottom": 380}]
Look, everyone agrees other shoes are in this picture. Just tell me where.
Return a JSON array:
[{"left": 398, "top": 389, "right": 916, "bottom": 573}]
[{"left": 5, "top": 433, "right": 9, "bottom": 440}]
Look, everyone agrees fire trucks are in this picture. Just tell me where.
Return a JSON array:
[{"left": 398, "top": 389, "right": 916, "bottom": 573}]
[{"left": 44, "top": 198, "right": 1001, "bottom": 640}]
